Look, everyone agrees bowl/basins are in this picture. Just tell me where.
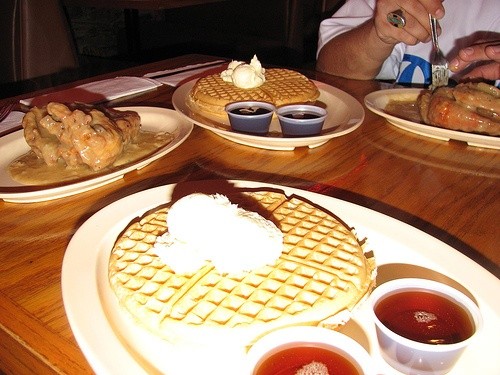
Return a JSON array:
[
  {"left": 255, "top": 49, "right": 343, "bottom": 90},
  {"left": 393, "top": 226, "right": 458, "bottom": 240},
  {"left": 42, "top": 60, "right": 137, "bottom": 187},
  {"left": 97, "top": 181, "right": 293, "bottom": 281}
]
[
  {"left": 368, "top": 278, "right": 482, "bottom": 375},
  {"left": 276, "top": 105, "right": 328, "bottom": 138},
  {"left": 242, "top": 325, "right": 374, "bottom": 375},
  {"left": 226, "top": 101, "right": 275, "bottom": 136}
]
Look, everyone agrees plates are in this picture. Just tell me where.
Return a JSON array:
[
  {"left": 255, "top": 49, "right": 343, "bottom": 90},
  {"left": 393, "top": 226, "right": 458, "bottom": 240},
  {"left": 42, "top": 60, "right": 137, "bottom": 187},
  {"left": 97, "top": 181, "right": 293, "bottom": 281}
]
[
  {"left": 61, "top": 180, "right": 500, "bottom": 375},
  {"left": 364, "top": 88, "right": 500, "bottom": 149},
  {"left": 0, "top": 106, "right": 193, "bottom": 204},
  {"left": 172, "top": 77, "right": 365, "bottom": 150}
]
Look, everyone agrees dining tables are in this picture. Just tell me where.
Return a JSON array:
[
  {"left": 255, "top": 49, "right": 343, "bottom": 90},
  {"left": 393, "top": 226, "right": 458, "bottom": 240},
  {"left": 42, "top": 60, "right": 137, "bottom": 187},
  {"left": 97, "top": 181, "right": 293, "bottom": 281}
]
[{"left": 0, "top": 54, "right": 500, "bottom": 375}]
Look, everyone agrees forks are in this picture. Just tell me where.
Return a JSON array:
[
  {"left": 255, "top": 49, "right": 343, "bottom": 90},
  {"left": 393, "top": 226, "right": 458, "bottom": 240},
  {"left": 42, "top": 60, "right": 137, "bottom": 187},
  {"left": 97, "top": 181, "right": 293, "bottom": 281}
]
[
  {"left": 429, "top": 13, "right": 449, "bottom": 91},
  {"left": 0, "top": 103, "right": 17, "bottom": 122}
]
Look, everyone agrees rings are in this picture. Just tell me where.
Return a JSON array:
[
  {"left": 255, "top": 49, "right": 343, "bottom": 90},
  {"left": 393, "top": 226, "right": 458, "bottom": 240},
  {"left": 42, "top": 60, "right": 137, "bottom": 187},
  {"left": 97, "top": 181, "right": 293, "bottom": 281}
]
[{"left": 387, "top": 13, "right": 405, "bottom": 28}]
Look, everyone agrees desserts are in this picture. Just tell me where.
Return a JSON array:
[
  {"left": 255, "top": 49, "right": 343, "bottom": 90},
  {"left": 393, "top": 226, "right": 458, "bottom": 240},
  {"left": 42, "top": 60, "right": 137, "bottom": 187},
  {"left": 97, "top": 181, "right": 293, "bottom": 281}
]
[
  {"left": 108, "top": 188, "right": 375, "bottom": 352},
  {"left": 188, "top": 53, "right": 320, "bottom": 118}
]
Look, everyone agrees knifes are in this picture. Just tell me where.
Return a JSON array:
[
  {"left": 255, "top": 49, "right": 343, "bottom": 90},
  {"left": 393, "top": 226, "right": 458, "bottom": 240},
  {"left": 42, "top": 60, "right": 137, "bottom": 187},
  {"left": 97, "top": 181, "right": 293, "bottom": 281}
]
[{"left": 149, "top": 60, "right": 228, "bottom": 79}]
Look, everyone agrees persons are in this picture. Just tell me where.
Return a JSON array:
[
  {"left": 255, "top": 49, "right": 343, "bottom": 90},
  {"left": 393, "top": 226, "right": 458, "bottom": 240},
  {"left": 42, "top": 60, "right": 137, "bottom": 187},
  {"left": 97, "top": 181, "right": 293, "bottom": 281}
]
[{"left": 315, "top": 0, "right": 500, "bottom": 89}]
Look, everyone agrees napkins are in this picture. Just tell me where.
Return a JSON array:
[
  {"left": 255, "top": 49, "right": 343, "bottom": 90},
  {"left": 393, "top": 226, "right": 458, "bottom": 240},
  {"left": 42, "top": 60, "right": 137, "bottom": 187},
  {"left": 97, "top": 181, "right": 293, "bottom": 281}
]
[
  {"left": 143, "top": 60, "right": 227, "bottom": 87},
  {"left": 0, "top": 111, "right": 27, "bottom": 133},
  {"left": 20, "top": 76, "right": 162, "bottom": 106}
]
